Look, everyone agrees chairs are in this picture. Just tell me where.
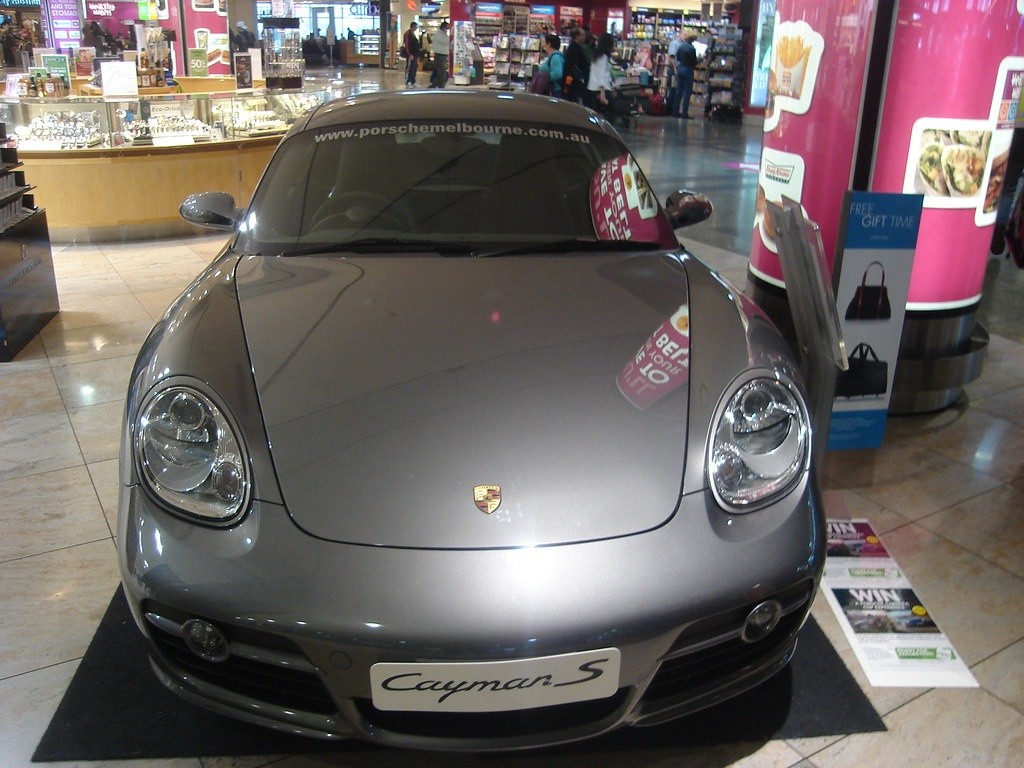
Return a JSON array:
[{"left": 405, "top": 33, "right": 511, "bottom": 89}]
[
  {"left": 326, "top": 130, "right": 412, "bottom": 232},
  {"left": 475, "top": 134, "right": 577, "bottom": 234}
]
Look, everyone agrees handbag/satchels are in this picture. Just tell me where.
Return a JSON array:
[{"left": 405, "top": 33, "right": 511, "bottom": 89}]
[
  {"left": 399, "top": 37, "right": 409, "bottom": 59},
  {"left": 528, "top": 72, "right": 549, "bottom": 95},
  {"left": 838, "top": 343, "right": 888, "bottom": 396},
  {"left": 644, "top": 92, "right": 664, "bottom": 116},
  {"left": 844, "top": 261, "right": 891, "bottom": 320}
]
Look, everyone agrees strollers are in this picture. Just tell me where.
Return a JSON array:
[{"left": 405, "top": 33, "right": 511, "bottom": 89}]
[{"left": 610, "top": 87, "right": 638, "bottom": 126}]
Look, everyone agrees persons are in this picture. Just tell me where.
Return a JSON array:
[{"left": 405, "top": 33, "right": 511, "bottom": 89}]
[
  {"left": 610, "top": 22, "right": 618, "bottom": 37},
  {"left": 237, "top": 56, "right": 251, "bottom": 88},
  {"left": 0, "top": 21, "right": 41, "bottom": 68},
  {"left": 229, "top": 21, "right": 258, "bottom": 76},
  {"left": 404, "top": 22, "right": 450, "bottom": 89},
  {"left": 666, "top": 31, "right": 703, "bottom": 119},
  {"left": 532, "top": 19, "right": 617, "bottom": 126},
  {"left": 420, "top": 29, "right": 432, "bottom": 62},
  {"left": 91, "top": 20, "right": 115, "bottom": 45},
  {"left": 302, "top": 27, "right": 355, "bottom": 67},
  {"left": 989, "top": 83, "right": 1024, "bottom": 255}
]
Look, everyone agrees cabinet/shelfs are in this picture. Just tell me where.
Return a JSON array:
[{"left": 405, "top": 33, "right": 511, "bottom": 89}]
[
  {"left": 449, "top": 0, "right": 744, "bottom": 120},
  {"left": 338, "top": 34, "right": 380, "bottom": 67},
  {"left": 0, "top": 123, "right": 60, "bottom": 363},
  {"left": 419, "top": 15, "right": 450, "bottom": 28},
  {"left": 263, "top": 17, "right": 306, "bottom": 89}
]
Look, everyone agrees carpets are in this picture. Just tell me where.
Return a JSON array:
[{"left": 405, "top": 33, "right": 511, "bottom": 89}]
[{"left": 31, "top": 580, "right": 889, "bottom": 763}]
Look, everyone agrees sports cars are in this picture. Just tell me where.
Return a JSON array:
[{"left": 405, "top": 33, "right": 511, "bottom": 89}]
[{"left": 117, "top": 88, "right": 824, "bottom": 755}]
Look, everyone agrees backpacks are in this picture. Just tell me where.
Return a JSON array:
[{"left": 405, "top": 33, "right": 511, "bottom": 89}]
[
  {"left": 548, "top": 52, "right": 585, "bottom": 94},
  {"left": 237, "top": 32, "right": 256, "bottom": 51}
]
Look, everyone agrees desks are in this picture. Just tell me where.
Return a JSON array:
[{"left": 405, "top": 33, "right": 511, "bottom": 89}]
[{"left": 78, "top": 84, "right": 179, "bottom": 97}]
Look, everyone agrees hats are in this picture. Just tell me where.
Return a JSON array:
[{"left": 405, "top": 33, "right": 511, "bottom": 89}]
[{"left": 235, "top": 21, "right": 248, "bottom": 30}]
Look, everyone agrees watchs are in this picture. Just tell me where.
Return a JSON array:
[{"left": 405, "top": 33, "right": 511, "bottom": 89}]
[
  {"left": 115, "top": 108, "right": 201, "bottom": 136},
  {"left": 245, "top": 98, "right": 269, "bottom": 129},
  {"left": 9, "top": 110, "right": 100, "bottom": 149}
]
